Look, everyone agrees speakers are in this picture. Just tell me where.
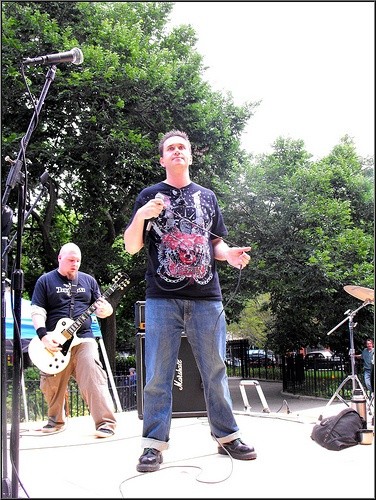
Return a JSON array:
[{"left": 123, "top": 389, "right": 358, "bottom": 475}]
[{"left": 136, "top": 332, "right": 207, "bottom": 420}]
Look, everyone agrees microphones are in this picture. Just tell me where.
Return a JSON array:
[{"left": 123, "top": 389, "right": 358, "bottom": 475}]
[
  {"left": 23, "top": 47, "right": 84, "bottom": 66},
  {"left": 145, "top": 193, "right": 163, "bottom": 236}
]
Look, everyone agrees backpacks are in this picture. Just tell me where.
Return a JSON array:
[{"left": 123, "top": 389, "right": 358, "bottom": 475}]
[{"left": 312, "top": 407, "right": 367, "bottom": 451}]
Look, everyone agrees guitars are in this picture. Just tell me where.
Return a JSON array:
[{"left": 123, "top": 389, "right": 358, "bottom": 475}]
[{"left": 27, "top": 272, "right": 131, "bottom": 375}]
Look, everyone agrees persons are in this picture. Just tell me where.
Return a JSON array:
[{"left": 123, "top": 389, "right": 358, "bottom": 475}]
[
  {"left": 125, "top": 368, "right": 136, "bottom": 390},
  {"left": 354, "top": 339, "right": 375, "bottom": 400},
  {"left": 31, "top": 243, "right": 116, "bottom": 437},
  {"left": 124, "top": 130, "right": 258, "bottom": 472}
]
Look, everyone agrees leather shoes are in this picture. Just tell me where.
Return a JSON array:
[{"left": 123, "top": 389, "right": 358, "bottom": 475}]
[
  {"left": 218, "top": 437, "right": 257, "bottom": 460},
  {"left": 137, "top": 447, "right": 163, "bottom": 472}
]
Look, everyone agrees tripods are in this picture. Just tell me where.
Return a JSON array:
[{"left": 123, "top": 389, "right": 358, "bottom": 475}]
[{"left": 318, "top": 298, "right": 374, "bottom": 425}]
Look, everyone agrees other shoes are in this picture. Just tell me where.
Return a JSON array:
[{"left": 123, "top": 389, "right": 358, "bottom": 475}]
[
  {"left": 41, "top": 424, "right": 64, "bottom": 431},
  {"left": 94, "top": 429, "right": 114, "bottom": 437}
]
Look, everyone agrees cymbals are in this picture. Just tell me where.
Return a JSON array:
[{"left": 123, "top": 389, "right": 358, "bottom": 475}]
[{"left": 343, "top": 284, "right": 375, "bottom": 306}]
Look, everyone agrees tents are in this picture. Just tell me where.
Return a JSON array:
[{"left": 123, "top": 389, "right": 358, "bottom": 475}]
[{"left": 5, "top": 290, "right": 122, "bottom": 423}]
